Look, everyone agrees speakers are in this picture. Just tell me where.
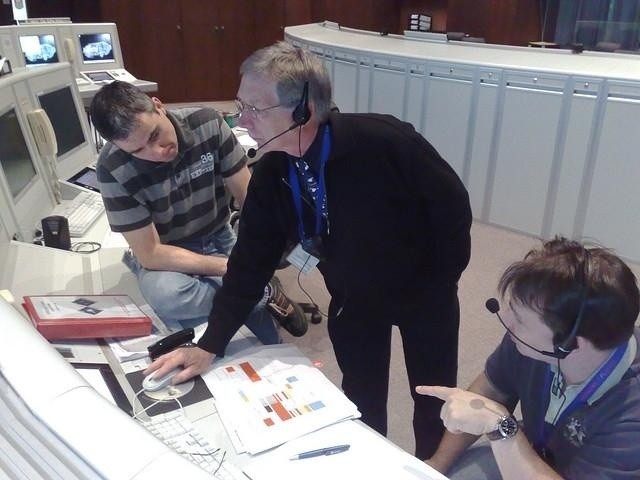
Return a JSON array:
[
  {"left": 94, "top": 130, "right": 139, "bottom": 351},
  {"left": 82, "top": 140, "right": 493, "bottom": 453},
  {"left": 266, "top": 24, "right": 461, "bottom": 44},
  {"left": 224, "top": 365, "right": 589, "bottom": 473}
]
[
  {"left": 11, "top": 0, "right": 28, "bottom": 20},
  {"left": 41, "top": 215, "right": 72, "bottom": 251}
]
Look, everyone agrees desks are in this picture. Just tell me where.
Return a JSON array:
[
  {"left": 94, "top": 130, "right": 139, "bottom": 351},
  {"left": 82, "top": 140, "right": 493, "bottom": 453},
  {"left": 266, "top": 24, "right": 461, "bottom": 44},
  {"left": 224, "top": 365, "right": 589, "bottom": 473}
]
[{"left": 53, "top": 213, "right": 444, "bottom": 480}]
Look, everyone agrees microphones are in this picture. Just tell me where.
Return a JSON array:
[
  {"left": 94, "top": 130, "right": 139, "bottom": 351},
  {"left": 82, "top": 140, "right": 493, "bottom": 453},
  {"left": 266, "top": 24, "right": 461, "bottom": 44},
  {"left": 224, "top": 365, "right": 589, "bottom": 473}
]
[
  {"left": 486, "top": 298, "right": 565, "bottom": 358},
  {"left": 248, "top": 123, "right": 299, "bottom": 158}
]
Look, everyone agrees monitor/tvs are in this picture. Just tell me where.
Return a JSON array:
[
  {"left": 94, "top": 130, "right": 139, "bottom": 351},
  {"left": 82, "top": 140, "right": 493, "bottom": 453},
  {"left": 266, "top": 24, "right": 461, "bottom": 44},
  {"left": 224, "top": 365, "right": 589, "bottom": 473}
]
[
  {"left": 67, "top": 167, "right": 101, "bottom": 194},
  {"left": 19, "top": 35, "right": 59, "bottom": 64},
  {"left": 83, "top": 72, "right": 114, "bottom": 81},
  {"left": 36, "top": 81, "right": 88, "bottom": 163},
  {"left": 79, "top": 33, "right": 115, "bottom": 64},
  {"left": 0, "top": 103, "right": 40, "bottom": 205}
]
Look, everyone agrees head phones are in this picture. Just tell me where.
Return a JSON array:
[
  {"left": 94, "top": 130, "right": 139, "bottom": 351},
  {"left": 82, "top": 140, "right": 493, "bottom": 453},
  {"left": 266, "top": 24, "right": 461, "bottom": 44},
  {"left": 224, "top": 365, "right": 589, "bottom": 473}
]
[
  {"left": 292, "top": 46, "right": 311, "bottom": 125},
  {"left": 553, "top": 249, "right": 593, "bottom": 359}
]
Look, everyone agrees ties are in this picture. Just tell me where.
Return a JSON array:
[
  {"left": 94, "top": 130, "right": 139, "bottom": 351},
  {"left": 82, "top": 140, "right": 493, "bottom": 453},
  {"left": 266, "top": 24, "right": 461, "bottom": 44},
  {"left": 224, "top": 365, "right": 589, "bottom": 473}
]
[{"left": 295, "top": 158, "right": 328, "bottom": 220}]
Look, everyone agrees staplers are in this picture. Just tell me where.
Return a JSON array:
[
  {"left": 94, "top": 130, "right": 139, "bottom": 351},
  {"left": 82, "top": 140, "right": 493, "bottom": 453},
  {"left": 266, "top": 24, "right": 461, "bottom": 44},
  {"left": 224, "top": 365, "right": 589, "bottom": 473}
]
[{"left": 147, "top": 328, "right": 196, "bottom": 362}]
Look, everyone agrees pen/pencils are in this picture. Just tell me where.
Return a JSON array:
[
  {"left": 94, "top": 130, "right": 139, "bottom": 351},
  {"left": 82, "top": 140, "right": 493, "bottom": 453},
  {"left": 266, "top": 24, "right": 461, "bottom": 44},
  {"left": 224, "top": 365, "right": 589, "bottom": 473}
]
[{"left": 289, "top": 445, "right": 351, "bottom": 461}]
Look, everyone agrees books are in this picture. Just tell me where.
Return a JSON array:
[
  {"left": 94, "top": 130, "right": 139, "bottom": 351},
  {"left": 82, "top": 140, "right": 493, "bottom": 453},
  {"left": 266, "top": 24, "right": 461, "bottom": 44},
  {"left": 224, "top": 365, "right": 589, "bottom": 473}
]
[{"left": 21, "top": 294, "right": 152, "bottom": 339}]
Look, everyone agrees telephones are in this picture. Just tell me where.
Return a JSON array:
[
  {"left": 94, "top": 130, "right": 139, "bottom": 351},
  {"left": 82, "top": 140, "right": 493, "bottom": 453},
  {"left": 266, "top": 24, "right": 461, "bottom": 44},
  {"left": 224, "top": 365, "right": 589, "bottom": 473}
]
[{"left": 27, "top": 108, "right": 59, "bottom": 158}]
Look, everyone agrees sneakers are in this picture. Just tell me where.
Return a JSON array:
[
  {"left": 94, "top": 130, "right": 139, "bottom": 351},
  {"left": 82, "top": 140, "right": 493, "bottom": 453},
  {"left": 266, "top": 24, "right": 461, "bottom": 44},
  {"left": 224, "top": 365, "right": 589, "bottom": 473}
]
[{"left": 264, "top": 276, "right": 308, "bottom": 337}]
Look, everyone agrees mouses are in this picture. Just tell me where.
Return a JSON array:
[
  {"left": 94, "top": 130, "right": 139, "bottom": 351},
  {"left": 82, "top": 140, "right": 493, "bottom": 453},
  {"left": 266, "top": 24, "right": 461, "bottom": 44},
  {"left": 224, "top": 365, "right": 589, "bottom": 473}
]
[{"left": 142, "top": 366, "right": 184, "bottom": 392}]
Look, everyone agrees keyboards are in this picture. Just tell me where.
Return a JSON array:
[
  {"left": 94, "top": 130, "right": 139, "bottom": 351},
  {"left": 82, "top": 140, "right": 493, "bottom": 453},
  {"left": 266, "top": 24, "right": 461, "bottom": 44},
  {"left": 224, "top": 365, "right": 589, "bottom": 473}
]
[
  {"left": 141, "top": 409, "right": 254, "bottom": 480},
  {"left": 62, "top": 191, "right": 106, "bottom": 237}
]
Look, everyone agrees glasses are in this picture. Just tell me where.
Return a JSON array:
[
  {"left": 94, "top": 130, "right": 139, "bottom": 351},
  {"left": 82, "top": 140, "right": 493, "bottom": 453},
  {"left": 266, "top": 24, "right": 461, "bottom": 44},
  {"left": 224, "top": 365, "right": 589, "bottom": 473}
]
[{"left": 234, "top": 100, "right": 301, "bottom": 119}]
